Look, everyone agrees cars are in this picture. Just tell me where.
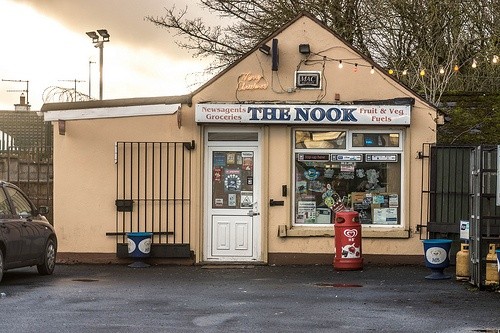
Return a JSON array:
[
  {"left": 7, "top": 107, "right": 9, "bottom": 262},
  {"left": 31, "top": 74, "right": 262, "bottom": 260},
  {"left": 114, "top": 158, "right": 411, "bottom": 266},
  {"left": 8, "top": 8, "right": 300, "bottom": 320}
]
[
  {"left": 0, "top": 182, "right": 58, "bottom": 283},
  {"left": 87, "top": 28, "right": 111, "bottom": 101}
]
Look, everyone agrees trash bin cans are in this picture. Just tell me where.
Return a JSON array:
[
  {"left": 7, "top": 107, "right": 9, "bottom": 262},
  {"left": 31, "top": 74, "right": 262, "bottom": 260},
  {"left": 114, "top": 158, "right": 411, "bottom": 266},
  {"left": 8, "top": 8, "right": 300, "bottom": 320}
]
[{"left": 332, "top": 211, "right": 364, "bottom": 272}]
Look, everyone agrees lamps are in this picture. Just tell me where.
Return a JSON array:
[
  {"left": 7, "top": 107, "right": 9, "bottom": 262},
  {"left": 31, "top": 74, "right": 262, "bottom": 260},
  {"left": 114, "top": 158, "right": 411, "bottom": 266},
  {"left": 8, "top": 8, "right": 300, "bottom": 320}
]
[
  {"left": 259, "top": 43, "right": 270, "bottom": 54},
  {"left": 298, "top": 43, "right": 311, "bottom": 57}
]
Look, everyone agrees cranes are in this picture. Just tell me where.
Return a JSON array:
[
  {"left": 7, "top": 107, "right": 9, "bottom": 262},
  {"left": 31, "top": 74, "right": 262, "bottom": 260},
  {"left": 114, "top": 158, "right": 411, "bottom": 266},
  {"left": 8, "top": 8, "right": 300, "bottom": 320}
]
[
  {"left": 2, "top": 79, "right": 30, "bottom": 111},
  {"left": 7, "top": 89, "right": 28, "bottom": 105},
  {"left": 59, "top": 79, "right": 84, "bottom": 102}
]
